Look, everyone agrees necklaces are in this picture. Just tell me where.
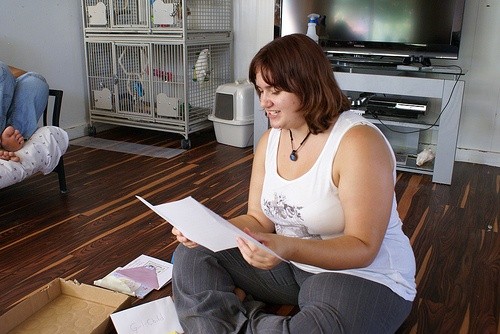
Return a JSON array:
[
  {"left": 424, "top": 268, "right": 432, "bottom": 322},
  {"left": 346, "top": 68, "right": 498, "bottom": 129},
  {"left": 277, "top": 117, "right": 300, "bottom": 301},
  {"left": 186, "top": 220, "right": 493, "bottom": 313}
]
[{"left": 289, "top": 129, "right": 312, "bottom": 161}]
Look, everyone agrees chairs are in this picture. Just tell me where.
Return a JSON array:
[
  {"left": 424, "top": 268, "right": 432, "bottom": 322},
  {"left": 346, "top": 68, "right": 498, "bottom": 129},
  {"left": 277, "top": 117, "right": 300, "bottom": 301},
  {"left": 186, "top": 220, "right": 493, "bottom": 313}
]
[{"left": 0, "top": 90, "right": 68, "bottom": 195}]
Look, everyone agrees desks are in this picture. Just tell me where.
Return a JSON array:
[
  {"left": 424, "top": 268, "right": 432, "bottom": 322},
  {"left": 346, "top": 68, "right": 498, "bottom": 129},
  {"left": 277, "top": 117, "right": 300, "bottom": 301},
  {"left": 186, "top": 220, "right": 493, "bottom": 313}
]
[{"left": 253, "top": 68, "right": 466, "bottom": 186}]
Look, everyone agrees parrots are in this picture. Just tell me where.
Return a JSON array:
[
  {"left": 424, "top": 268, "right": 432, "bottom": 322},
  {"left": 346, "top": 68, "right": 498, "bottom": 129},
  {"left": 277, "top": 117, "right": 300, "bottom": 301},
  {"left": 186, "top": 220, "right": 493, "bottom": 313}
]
[{"left": 195, "top": 49, "right": 211, "bottom": 84}]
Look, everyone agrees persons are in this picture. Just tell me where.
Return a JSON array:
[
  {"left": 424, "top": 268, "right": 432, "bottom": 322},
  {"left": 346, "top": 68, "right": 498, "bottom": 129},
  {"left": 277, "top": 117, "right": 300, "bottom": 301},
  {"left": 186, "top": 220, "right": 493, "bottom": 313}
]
[
  {"left": 0, "top": 63, "right": 50, "bottom": 162},
  {"left": 172, "top": 34, "right": 417, "bottom": 334}
]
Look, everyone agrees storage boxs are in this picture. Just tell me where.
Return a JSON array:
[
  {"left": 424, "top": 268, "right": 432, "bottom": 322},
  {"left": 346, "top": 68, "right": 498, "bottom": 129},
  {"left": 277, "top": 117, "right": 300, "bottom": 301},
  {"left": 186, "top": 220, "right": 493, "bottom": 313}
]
[
  {"left": 207, "top": 79, "right": 252, "bottom": 148},
  {"left": 0, "top": 277, "right": 129, "bottom": 334}
]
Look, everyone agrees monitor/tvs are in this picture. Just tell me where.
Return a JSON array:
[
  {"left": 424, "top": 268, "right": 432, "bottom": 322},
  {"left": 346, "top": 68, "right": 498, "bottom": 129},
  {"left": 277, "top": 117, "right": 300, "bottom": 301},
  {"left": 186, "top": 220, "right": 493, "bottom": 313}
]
[{"left": 273, "top": 0, "right": 465, "bottom": 60}]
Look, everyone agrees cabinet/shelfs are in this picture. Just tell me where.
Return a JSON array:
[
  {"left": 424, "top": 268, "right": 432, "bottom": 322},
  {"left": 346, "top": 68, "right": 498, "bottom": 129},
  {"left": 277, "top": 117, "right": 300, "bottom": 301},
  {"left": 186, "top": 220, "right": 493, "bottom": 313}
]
[{"left": 80, "top": 0, "right": 234, "bottom": 150}]
